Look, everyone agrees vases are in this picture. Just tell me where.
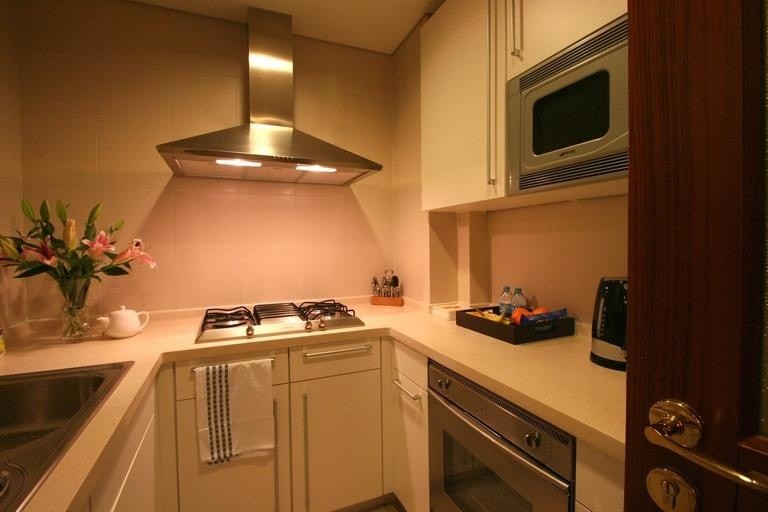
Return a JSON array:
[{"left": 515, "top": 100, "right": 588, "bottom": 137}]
[{"left": 52, "top": 276, "right": 97, "bottom": 340}]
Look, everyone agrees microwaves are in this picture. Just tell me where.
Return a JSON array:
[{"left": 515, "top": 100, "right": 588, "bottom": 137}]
[{"left": 504, "top": 13, "right": 633, "bottom": 197}]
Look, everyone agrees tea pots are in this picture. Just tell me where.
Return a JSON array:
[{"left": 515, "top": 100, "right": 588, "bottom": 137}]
[{"left": 97, "top": 305, "right": 152, "bottom": 340}]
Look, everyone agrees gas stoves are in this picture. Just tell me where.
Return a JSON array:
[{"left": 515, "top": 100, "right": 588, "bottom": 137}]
[{"left": 194, "top": 299, "right": 366, "bottom": 343}]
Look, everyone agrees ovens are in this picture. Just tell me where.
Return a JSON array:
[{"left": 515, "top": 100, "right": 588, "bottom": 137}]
[{"left": 427, "top": 357, "right": 576, "bottom": 512}]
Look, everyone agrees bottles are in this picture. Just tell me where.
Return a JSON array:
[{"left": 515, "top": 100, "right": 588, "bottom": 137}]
[
  {"left": 371, "top": 269, "right": 401, "bottom": 297},
  {"left": 498, "top": 286, "right": 526, "bottom": 319}
]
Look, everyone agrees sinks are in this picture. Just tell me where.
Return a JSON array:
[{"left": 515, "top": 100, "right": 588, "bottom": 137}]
[{"left": 0, "top": 360, "right": 136, "bottom": 452}]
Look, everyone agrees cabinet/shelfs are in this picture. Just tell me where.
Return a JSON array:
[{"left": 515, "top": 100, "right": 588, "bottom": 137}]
[
  {"left": 507, "top": 2, "right": 631, "bottom": 82},
  {"left": 419, "top": 0, "right": 505, "bottom": 214},
  {"left": 388, "top": 365, "right": 431, "bottom": 510},
  {"left": 174, "top": 343, "right": 382, "bottom": 511}
]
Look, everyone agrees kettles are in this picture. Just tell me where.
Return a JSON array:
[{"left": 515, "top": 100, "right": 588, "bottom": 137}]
[{"left": 589, "top": 276, "right": 627, "bottom": 371}]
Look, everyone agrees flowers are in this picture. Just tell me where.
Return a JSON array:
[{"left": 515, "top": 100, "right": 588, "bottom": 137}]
[{"left": 0, "top": 197, "right": 156, "bottom": 341}]
